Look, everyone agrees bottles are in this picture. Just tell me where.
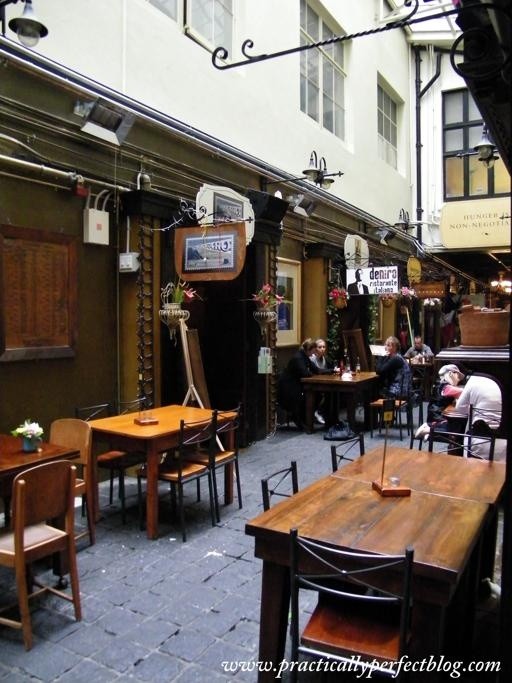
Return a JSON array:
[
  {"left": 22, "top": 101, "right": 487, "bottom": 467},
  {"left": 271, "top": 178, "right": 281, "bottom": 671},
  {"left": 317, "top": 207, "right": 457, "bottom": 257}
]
[{"left": 334, "top": 355, "right": 361, "bottom": 377}]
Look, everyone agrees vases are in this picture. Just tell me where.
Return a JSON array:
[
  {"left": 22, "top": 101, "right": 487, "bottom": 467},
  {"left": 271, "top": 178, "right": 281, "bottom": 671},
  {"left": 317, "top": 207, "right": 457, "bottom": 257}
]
[
  {"left": 158, "top": 302, "right": 190, "bottom": 335},
  {"left": 253, "top": 308, "right": 276, "bottom": 328},
  {"left": 400, "top": 306, "right": 409, "bottom": 314},
  {"left": 334, "top": 300, "right": 348, "bottom": 310}
]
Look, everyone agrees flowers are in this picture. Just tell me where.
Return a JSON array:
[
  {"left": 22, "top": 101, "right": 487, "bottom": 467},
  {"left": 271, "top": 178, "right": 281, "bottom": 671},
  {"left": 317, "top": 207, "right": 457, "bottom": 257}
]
[
  {"left": 250, "top": 282, "right": 283, "bottom": 307},
  {"left": 330, "top": 285, "right": 351, "bottom": 300},
  {"left": 378, "top": 285, "right": 418, "bottom": 306},
  {"left": 163, "top": 278, "right": 196, "bottom": 303}
]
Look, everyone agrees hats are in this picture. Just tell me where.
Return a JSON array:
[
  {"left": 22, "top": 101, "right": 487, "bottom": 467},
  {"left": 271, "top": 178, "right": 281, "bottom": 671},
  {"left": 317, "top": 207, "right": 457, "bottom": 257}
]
[{"left": 438, "top": 364, "right": 465, "bottom": 380}]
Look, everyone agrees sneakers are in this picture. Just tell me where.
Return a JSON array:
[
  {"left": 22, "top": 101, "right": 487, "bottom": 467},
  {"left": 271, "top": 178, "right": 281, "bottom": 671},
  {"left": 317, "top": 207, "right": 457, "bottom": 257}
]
[
  {"left": 314, "top": 410, "right": 325, "bottom": 424},
  {"left": 414, "top": 422, "right": 430, "bottom": 442}
]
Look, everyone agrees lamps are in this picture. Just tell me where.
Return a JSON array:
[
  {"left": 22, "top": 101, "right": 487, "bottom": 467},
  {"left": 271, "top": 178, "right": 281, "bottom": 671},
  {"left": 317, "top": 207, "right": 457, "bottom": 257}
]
[
  {"left": 472, "top": 121, "right": 499, "bottom": 169},
  {"left": 303, "top": 150, "right": 335, "bottom": 190},
  {"left": 393, "top": 207, "right": 415, "bottom": 234},
  {"left": 0, "top": 0, "right": 49, "bottom": 48}
]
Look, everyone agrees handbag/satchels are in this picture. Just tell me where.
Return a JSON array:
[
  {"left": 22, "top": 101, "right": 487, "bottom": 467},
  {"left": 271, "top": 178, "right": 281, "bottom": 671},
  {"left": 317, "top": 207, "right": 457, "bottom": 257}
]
[{"left": 323, "top": 420, "right": 355, "bottom": 440}]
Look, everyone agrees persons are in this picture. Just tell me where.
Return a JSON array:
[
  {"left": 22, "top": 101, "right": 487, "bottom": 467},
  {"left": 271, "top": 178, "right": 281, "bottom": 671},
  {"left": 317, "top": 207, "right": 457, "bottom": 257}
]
[
  {"left": 448, "top": 363, "right": 503, "bottom": 454},
  {"left": 277, "top": 337, "right": 342, "bottom": 428},
  {"left": 309, "top": 339, "right": 339, "bottom": 423},
  {"left": 404, "top": 334, "right": 434, "bottom": 359},
  {"left": 348, "top": 268, "right": 369, "bottom": 294},
  {"left": 415, "top": 363, "right": 464, "bottom": 442},
  {"left": 362, "top": 336, "right": 413, "bottom": 431}
]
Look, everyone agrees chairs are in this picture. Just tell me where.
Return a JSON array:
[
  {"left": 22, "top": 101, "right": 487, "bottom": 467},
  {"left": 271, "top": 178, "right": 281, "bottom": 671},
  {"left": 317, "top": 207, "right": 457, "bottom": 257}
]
[{"left": 277, "top": 352, "right": 423, "bottom": 440}]
